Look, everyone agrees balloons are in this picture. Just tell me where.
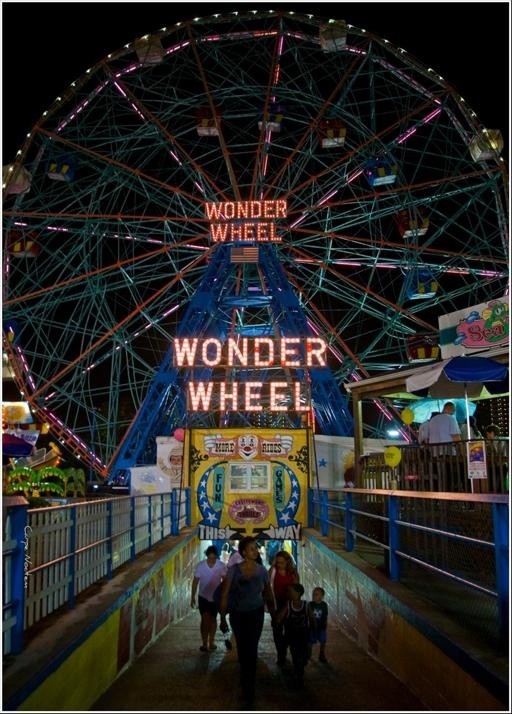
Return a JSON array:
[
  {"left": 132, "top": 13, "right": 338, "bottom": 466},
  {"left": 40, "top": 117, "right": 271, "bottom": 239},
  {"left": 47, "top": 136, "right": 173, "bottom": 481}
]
[
  {"left": 384, "top": 446, "right": 401, "bottom": 471},
  {"left": 400, "top": 408, "right": 415, "bottom": 425},
  {"left": 173, "top": 428, "right": 184, "bottom": 441}
]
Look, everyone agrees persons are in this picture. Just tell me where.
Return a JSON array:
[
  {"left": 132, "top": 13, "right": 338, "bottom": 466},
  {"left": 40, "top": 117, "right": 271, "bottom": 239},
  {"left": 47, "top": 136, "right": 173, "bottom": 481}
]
[
  {"left": 190, "top": 536, "right": 329, "bottom": 708},
  {"left": 416, "top": 401, "right": 508, "bottom": 509}
]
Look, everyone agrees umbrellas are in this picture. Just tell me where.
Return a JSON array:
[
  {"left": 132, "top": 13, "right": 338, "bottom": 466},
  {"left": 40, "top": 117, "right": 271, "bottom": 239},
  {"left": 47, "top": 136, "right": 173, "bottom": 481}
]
[{"left": 405, "top": 354, "right": 509, "bottom": 441}]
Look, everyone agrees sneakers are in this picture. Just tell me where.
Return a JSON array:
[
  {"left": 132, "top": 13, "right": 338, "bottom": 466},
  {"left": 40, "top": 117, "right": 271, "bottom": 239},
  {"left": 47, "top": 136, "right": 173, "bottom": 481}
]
[
  {"left": 209, "top": 645, "right": 217, "bottom": 649},
  {"left": 199, "top": 646, "right": 208, "bottom": 652},
  {"left": 318, "top": 654, "right": 326, "bottom": 662}
]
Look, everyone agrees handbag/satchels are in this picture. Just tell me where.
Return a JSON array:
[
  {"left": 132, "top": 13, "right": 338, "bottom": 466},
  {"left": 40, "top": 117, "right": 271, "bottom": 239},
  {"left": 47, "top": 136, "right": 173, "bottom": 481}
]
[{"left": 264, "top": 591, "right": 277, "bottom": 612}]
